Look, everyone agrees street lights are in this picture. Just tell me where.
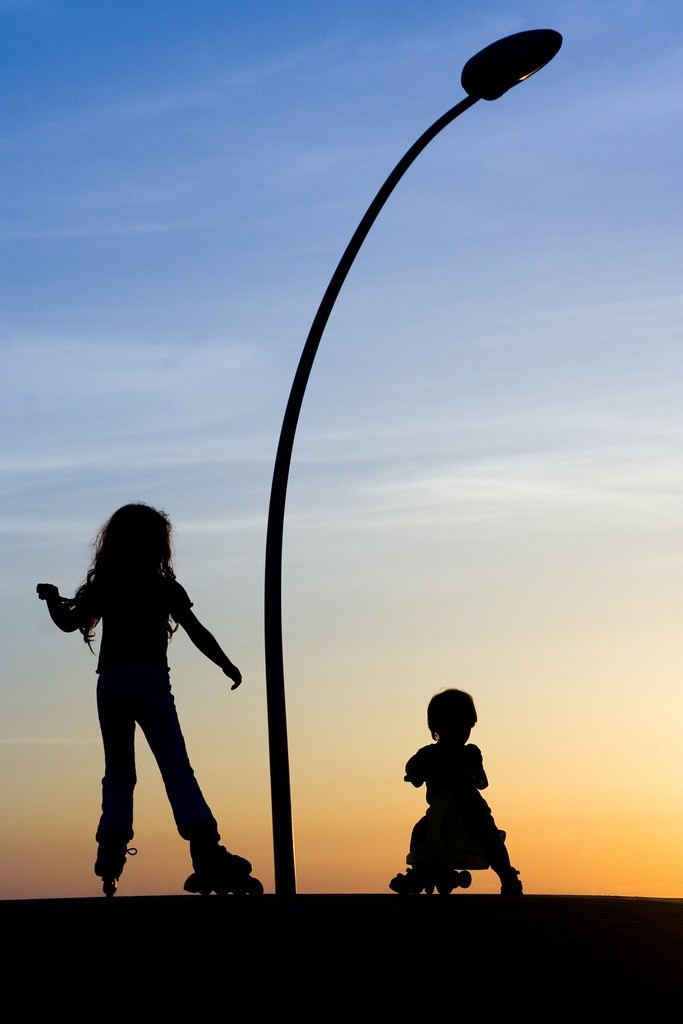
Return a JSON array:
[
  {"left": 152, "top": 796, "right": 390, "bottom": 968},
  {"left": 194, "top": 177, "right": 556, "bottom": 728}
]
[{"left": 261, "top": 29, "right": 565, "bottom": 891}]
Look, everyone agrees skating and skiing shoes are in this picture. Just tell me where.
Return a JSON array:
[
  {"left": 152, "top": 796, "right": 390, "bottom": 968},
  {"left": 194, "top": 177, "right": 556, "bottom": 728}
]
[
  {"left": 94, "top": 844, "right": 137, "bottom": 895},
  {"left": 184, "top": 846, "right": 265, "bottom": 895}
]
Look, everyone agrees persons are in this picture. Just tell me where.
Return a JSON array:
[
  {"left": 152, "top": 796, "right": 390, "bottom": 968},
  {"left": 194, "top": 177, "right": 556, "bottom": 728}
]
[
  {"left": 389, "top": 688, "right": 524, "bottom": 894},
  {"left": 36, "top": 504, "right": 252, "bottom": 877}
]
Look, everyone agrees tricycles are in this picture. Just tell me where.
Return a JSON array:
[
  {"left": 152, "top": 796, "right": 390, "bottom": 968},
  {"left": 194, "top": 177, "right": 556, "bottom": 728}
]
[{"left": 393, "top": 796, "right": 489, "bottom": 895}]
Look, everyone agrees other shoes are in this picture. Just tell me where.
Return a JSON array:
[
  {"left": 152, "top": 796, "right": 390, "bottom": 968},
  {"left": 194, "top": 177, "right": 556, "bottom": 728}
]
[
  {"left": 389, "top": 868, "right": 423, "bottom": 893},
  {"left": 500, "top": 866, "right": 523, "bottom": 895}
]
